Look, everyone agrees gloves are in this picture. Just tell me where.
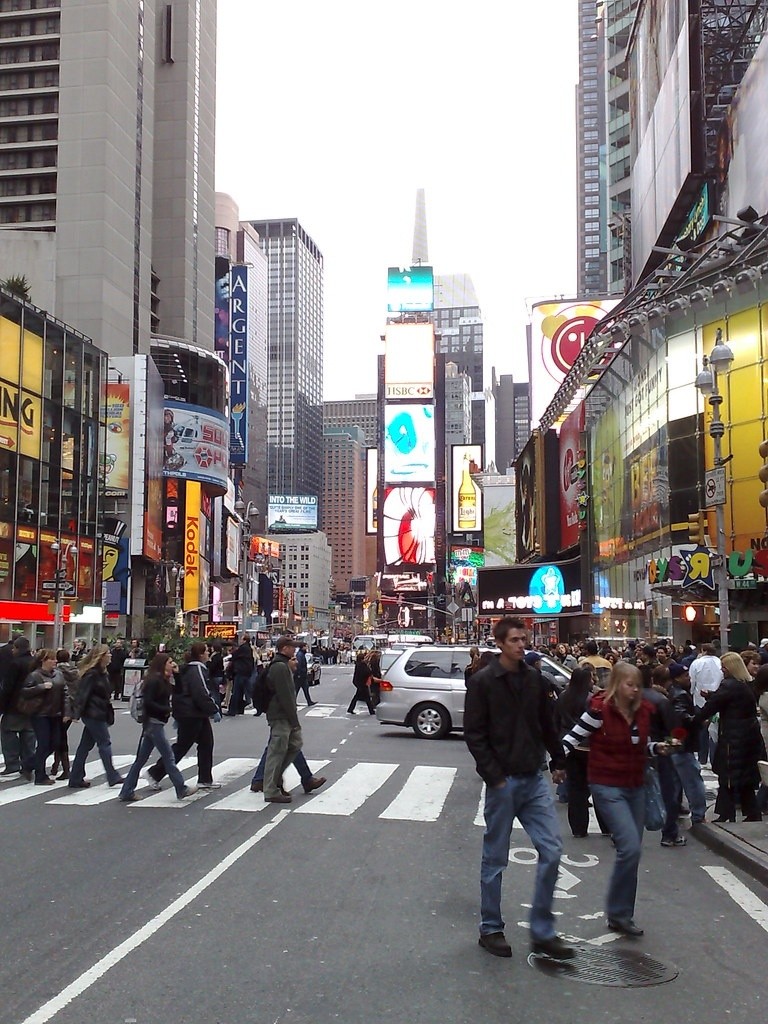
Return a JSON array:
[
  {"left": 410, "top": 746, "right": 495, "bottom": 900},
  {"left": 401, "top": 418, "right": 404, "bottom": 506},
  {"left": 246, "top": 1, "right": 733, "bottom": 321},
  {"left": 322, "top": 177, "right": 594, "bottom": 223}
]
[
  {"left": 212, "top": 712, "right": 221, "bottom": 723},
  {"left": 172, "top": 720, "right": 178, "bottom": 730}
]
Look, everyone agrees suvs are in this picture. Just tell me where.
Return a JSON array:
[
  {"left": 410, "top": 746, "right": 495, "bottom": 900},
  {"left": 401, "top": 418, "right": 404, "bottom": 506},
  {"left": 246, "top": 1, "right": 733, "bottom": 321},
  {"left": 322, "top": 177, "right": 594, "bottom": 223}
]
[{"left": 375, "top": 645, "right": 597, "bottom": 739}]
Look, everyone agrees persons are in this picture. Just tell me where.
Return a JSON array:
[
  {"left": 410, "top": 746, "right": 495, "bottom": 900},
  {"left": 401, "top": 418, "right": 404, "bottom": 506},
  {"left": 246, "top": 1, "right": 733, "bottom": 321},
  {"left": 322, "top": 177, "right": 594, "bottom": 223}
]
[
  {"left": 347, "top": 650, "right": 382, "bottom": 716},
  {"left": 549, "top": 660, "right": 676, "bottom": 935},
  {"left": 0, "top": 634, "right": 350, "bottom": 803},
  {"left": 162, "top": 409, "right": 185, "bottom": 471},
  {"left": 524, "top": 639, "right": 768, "bottom": 846},
  {"left": 465, "top": 646, "right": 496, "bottom": 683},
  {"left": 463, "top": 616, "right": 576, "bottom": 959}
]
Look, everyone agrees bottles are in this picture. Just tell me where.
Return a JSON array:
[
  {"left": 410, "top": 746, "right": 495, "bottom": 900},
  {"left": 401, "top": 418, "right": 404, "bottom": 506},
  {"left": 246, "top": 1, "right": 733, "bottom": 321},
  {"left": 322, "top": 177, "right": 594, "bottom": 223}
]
[{"left": 135, "top": 698, "right": 144, "bottom": 722}]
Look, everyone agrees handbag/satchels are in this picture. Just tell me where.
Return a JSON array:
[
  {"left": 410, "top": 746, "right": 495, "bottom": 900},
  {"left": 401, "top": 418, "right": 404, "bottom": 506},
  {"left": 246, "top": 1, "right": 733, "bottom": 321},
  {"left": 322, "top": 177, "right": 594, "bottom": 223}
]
[
  {"left": 128, "top": 680, "right": 144, "bottom": 724},
  {"left": 644, "top": 769, "right": 668, "bottom": 831},
  {"left": 106, "top": 703, "right": 114, "bottom": 725},
  {"left": 17, "top": 671, "right": 48, "bottom": 717}
]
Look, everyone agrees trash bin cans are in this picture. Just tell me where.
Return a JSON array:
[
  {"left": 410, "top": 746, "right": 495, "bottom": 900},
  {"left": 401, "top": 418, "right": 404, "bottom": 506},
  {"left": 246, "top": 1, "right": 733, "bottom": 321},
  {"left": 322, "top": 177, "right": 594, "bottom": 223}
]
[{"left": 120, "top": 658, "right": 149, "bottom": 702}]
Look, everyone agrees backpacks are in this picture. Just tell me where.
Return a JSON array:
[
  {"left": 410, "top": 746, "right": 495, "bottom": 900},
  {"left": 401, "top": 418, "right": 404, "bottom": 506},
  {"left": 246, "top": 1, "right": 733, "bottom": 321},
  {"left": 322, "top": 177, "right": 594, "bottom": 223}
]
[{"left": 251, "top": 659, "right": 291, "bottom": 712}]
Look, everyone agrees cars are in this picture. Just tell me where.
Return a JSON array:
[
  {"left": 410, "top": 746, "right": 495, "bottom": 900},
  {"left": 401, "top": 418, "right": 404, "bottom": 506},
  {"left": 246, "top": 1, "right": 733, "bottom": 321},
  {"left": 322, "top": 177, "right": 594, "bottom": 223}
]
[
  {"left": 292, "top": 653, "right": 322, "bottom": 686},
  {"left": 351, "top": 634, "right": 435, "bottom": 675}
]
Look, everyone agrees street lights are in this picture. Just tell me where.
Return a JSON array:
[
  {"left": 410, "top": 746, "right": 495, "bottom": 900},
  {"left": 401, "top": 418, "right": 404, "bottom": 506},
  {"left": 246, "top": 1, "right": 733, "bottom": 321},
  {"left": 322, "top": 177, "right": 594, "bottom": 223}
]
[
  {"left": 51, "top": 540, "right": 60, "bottom": 662},
  {"left": 59, "top": 541, "right": 79, "bottom": 651},
  {"left": 694, "top": 328, "right": 736, "bottom": 658},
  {"left": 234, "top": 496, "right": 259, "bottom": 644}
]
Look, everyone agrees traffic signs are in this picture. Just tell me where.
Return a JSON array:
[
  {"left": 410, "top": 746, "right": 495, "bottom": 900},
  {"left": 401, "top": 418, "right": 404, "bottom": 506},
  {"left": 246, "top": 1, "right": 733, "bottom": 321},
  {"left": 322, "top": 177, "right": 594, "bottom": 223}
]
[{"left": 42, "top": 579, "right": 74, "bottom": 593}]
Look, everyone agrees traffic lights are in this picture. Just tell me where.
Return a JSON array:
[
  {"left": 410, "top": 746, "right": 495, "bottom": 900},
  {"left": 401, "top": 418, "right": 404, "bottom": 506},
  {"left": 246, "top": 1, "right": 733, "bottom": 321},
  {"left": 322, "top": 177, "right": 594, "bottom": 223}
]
[
  {"left": 686, "top": 606, "right": 704, "bottom": 623},
  {"left": 756, "top": 441, "right": 768, "bottom": 512}
]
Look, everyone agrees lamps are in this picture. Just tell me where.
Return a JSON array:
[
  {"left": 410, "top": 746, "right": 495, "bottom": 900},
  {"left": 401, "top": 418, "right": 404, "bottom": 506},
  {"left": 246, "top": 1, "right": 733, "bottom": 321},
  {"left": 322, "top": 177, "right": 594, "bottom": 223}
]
[{"left": 109, "top": 366, "right": 123, "bottom": 385}]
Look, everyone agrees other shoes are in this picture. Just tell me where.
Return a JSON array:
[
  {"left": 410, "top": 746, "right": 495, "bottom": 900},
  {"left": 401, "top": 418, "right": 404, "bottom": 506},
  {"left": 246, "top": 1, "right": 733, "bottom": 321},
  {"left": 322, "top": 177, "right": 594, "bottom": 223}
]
[
  {"left": 114, "top": 697, "right": 120, "bottom": 700},
  {"left": 531, "top": 939, "right": 575, "bottom": 959},
  {"left": 559, "top": 796, "right": 768, "bottom": 845},
  {"left": 478, "top": 932, "right": 512, "bottom": 957},
  {"left": 221, "top": 699, "right": 381, "bottom": 716},
  {"left": 608, "top": 920, "right": 644, "bottom": 936},
  {"left": 0, "top": 754, "right": 326, "bottom": 804}
]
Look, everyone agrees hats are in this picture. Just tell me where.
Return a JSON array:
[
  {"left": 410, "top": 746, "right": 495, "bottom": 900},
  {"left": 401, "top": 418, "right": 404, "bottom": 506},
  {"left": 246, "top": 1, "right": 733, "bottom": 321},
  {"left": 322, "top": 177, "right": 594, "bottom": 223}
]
[
  {"left": 14, "top": 636, "right": 30, "bottom": 649},
  {"left": 276, "top": 636, "right": 301, "bottom": 648},
  {"left": 669, "top": 663, "right": 689, "bottom": 679},
  {"left": 639, "top": 644, "right": 655, "bottom": 657},
  {"left": 760, "top": 638, "right": 768, "bottom": 648},
  {"left": 525, "top": 652, "right": 546, "bottom": 664}
]
